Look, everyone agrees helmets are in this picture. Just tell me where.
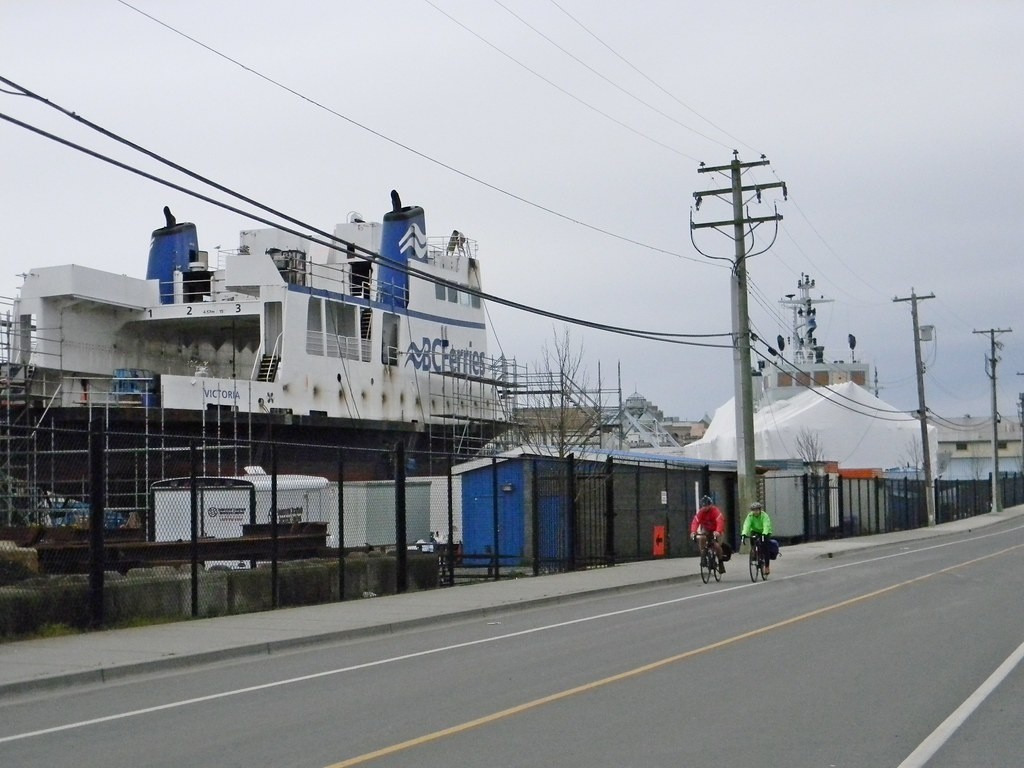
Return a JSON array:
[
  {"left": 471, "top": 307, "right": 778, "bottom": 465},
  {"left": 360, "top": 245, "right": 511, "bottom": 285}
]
[
  {"left": 750, "top": 502, "right": 761, "bottom": 510},
  {"left": 699, "top": 495, "right": 712, "bottom": 506}
]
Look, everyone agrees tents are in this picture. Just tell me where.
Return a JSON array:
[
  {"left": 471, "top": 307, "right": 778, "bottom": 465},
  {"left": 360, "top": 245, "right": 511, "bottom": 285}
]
[{"left": 684, "top": 381, "right": 939, "bottom": 472}]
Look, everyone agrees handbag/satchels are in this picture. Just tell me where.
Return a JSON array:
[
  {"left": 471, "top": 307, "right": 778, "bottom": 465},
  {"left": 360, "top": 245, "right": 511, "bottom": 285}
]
[
  {"left": 769, "top": 539, "right": 779, "bottom": 560},
  {"left": 722, "top": 542, "right": 734, "bottom": 561}
]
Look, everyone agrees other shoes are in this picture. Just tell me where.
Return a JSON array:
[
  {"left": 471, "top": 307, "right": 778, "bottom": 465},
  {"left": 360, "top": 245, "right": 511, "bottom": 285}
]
[
  {"left": 700, "top": 559, "right": 707, "bottom": 567},
  {"left": 765, "top": 566, "right": 770, "bottom": 575},
  {"left": 719, "top": 560, "right": 726, "bottom": 574}
]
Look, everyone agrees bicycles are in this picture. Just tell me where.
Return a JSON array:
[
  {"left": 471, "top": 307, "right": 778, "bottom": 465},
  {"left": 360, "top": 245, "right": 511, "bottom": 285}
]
[
  {"left": 691, "top": 531, "right": 724, "bottom": 584},
  {"left": 740, "top": 532, "right": 770, "bottom": 583}
]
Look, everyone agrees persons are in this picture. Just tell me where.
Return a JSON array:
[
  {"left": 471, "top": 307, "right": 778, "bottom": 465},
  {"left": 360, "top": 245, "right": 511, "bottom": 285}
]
[
  {"left": 741, "top": 502, "right": 772, "bottom": 574},
  {"left": 690, "top": 495, "right": 726, "bottom": 574}
]
